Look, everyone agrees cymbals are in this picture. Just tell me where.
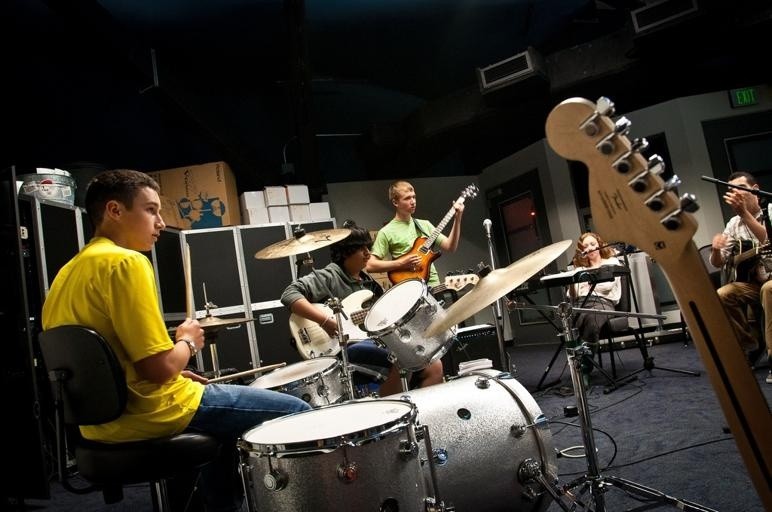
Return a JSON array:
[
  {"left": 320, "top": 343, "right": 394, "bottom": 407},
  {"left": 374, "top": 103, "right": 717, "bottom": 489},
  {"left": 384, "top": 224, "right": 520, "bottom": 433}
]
[
  {"left": 423, "top": 237, "right": 572, "bottom": 341},
  {"left": 168, "top": 316, "right": 259, "bottom": 334},
  {"left": 254, "top": 227, "right": 352, "bottom": 260}
]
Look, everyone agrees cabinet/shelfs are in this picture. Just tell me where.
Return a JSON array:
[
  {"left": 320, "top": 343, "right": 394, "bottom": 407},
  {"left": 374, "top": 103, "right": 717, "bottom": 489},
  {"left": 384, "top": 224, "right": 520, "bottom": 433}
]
[
  {"left": 183, "top": 218, "right": 338, "bottom": 385},
  {"left": 32, "top": 199, "right": 209, "bottom": 377}
]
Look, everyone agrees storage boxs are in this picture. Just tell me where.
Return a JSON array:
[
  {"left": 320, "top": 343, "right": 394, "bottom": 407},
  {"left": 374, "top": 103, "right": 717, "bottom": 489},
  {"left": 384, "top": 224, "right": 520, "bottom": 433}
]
[
  {"left": 287, "top": 185, "right": 310, "bottom": 204},
  {"left": 146, "top": 160, "right": 241, "bottom": 230},
  {"left": 241, "top": 192, "right": 265, "bottom": 209},
  {"left": 242, "top": 210, "right": 269, "bottom": 224},
  {"left": 267, "top": 206, "right": 290, "bottom": 223},
  {"left": 264, "top": 186, "right": 287, "bottom": 205},
  {"left": 309, "top": 202, "right": 332, "bottom": 221},
  {"left": 289, "top": 205, "right": 310, "bottom": 222}
]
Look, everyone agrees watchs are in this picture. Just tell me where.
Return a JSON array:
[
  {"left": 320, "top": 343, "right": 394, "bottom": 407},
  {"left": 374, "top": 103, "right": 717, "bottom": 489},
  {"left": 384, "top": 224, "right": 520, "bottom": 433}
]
[{"left": 175, "top": 336, "right": 199, "bottom": 357}]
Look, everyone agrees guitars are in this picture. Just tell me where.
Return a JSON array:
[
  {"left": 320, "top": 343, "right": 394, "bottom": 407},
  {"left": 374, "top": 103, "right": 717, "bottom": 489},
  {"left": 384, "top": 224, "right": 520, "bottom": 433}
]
[
  {"left": 387, "top": 183, "right": 479, "bottom": 283},
  {"left": 288, "top": 274, "right": 479, "bottom": 359},
  {"left": 545, "top": 96, "right": 771, "bottom": 510},
  {"left": 698, "top": 239, "right": 772, "bottom": 290}
]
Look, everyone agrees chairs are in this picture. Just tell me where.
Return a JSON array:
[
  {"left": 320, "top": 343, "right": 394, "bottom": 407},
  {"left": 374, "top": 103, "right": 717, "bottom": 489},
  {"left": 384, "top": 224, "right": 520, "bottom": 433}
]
[
  {"left": 598, "top": 256, "right": 654, "bottom": 380},
  {"left": 39, "top": 325, "right": 221, "bottom": 511}
]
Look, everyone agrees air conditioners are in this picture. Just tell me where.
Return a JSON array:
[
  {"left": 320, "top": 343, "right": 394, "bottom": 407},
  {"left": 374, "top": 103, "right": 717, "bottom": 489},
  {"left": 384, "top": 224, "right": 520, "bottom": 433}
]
[
  {"left": 630, "top": 0, "right": 699, "bottom": 34},
  {"left": 475, "top": 45, "right": 551, "bottom": 96}
]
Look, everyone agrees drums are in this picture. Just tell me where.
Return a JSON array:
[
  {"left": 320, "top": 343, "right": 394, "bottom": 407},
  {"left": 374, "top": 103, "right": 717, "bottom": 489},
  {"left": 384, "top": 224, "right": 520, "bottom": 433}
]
[
  {"left": 364, "top": 277, "right": 459, "bottom": 372},
  {"left": 248, "top": 357, "right": 348, "bottom": 411},
  {"left": 380, "top": 368, "right": 560, "bottom": 511},
  {"left": 235, "top": 399, "right": 456, "bottom": 512}
]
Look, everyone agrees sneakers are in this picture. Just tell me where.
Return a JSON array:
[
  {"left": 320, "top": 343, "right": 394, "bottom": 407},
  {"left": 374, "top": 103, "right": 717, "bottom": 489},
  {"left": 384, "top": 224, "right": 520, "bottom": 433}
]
[{"left": 766, "top": 370, "right": 772, "bottom": 384}]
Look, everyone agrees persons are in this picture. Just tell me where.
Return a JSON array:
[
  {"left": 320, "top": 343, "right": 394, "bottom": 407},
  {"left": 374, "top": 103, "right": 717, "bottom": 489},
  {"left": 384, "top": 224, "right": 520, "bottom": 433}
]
[
  {"left": 565, "top": 232, "right": 622, "bottom": 390},
  {"left": 709, "top": 172, "right": 772, "bottom": 383},
  {"left": 280, "top": 227, "right": 443, "bottom": 398},
  {"left": 366, "top": 181, "right": 465, "bottom": 306},
  {"left": 41, "top": 169, "right": 313, "bottom": 512}
]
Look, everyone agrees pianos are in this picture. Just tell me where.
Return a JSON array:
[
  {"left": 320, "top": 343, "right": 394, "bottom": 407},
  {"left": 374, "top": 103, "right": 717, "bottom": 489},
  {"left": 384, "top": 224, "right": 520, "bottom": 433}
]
[{"left": 505, "top": 264, "right": 629, "bottom": 299}]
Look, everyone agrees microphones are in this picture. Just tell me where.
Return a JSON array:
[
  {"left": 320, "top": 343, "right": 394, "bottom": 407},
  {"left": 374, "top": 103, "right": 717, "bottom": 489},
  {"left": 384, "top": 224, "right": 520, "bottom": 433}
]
[{"left": 481, "top": 218, "right": 494, "bottom": 237}]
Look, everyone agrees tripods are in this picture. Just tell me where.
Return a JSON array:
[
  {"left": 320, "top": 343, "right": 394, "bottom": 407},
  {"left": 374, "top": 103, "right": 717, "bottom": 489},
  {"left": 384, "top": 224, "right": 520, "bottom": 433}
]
[
  {"left": 505, "top": 294, "right": 715, "bottom": 511},
  {"left": 582, "top": 241, "right": 702, "bottom": 386}
]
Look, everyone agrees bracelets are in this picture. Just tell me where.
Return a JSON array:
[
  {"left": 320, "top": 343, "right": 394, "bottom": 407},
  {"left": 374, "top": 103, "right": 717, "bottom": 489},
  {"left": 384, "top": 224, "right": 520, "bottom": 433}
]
[{"left": 320, "top": 316, "right": 329, "bottom": 328}]
